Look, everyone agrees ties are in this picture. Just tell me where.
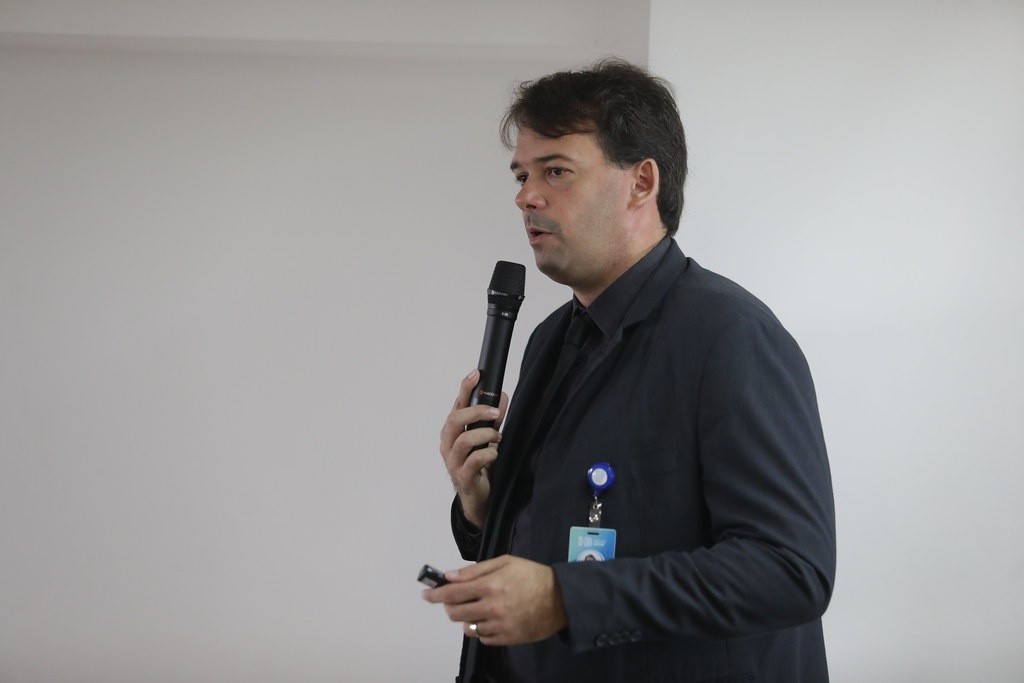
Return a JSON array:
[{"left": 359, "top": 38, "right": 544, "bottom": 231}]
[{"left": 485, "top": 316, "right": 593, "bottom": 555}]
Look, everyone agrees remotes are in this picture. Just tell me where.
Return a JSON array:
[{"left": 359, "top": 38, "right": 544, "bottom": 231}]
[{"left": 417, "top": 565, "right": 449, "bottom": 588}]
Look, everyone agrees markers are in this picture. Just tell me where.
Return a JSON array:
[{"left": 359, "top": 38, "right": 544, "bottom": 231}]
[{"left": 415, "top": 564, "right": 451, "bottom": 589}]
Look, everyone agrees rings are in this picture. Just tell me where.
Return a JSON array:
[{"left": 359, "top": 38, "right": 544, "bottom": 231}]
[{"left": 468, "top": 623, "right": 480, "bottom": 639}]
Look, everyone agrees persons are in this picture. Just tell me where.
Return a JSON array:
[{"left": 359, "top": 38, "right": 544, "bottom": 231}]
[{"left": 421, "top": 55, "right": 837, "bottom": 683}]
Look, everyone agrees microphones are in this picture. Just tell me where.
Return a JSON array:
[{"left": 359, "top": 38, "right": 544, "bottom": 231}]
[{"left": 466, "top": 259, "right": 526, "bottom": 450}]
